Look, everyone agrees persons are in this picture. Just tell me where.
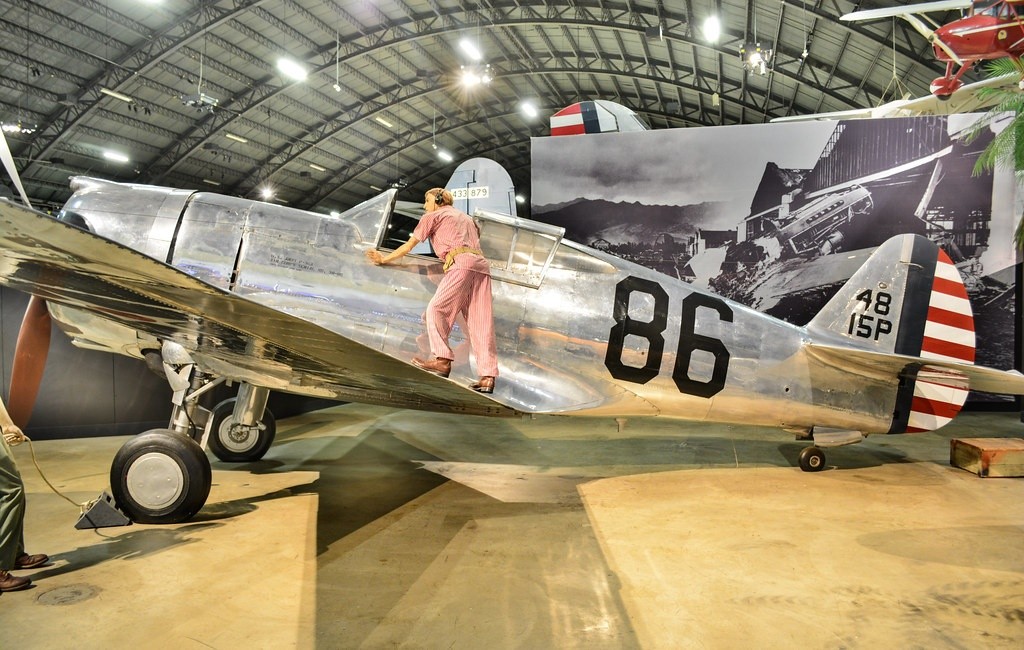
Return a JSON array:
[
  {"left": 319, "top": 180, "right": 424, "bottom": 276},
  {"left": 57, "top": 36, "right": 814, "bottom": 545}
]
[
  {"left": 367, "top": 187, "right": 499, "bottom": 394},
  {"left": 0, "top": 397, "right": 49, "bottom": 592}
]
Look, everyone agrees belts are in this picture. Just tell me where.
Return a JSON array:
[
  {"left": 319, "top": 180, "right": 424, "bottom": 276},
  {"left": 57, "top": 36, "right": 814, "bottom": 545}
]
[{"left": 443, "top": 248, "right": 481, "bottom": 270}]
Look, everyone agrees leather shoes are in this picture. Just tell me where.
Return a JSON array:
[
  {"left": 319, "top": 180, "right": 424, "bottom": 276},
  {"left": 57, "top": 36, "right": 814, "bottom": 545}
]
[
  {"left": 0, "top": 571, "right": 32, "bottom": 592},
  {"left": 411, "top": 355, "right": 451, "bottom": 379},
  {"left": 14, "top": 553, "right": 49, "bottom": 568},
  {"left": 471, "top": 376, "right": 494, "bottom": 394}
]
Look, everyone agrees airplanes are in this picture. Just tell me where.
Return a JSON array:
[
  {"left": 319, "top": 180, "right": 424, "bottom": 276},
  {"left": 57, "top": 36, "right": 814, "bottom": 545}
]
[
  {"left": 839, "top": 1, "right": 1024, "bottom": 101},
  {"left": 1, "top": 155, "right": 1024, "bottom": 524},
  {"left": 682, "top": 146, "right": 955, "bottom": 314}
]
